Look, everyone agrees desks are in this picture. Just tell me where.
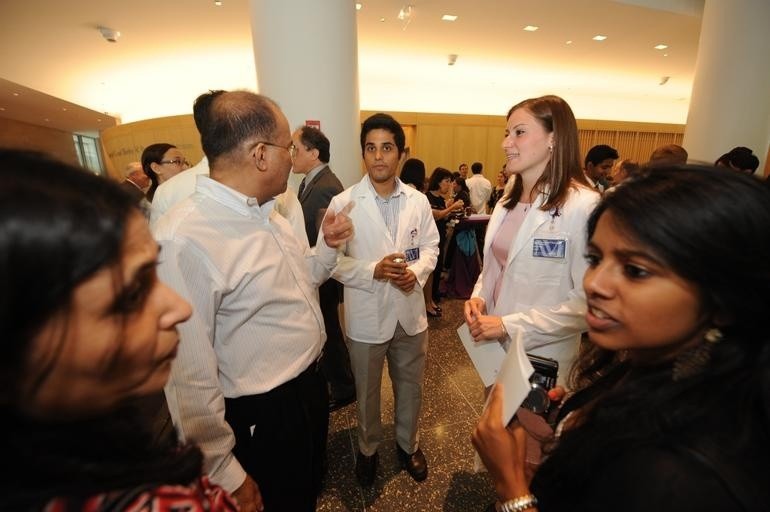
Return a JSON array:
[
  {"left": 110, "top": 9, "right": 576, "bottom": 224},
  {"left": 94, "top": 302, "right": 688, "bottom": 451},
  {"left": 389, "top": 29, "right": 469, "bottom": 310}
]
[{"left": 448, "top": 210, "right": 496, "bottom": 301}]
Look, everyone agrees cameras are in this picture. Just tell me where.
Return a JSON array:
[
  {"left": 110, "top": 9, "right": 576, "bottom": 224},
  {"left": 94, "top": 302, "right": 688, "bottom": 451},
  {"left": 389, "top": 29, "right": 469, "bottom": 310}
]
[{"left": 520, "top": 353, "right": 559, "bottom": 423}]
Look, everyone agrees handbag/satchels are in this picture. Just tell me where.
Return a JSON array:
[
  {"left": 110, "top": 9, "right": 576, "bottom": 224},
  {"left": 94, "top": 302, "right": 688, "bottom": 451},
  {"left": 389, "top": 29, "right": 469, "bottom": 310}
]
[{"left": 44, "top": 473, "right": 243, "bottom": 512}]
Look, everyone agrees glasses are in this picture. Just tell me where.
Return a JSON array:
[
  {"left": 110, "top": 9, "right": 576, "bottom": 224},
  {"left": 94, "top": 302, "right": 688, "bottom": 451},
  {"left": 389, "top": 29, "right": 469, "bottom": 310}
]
[
  {"left": 155, "top": 158, "right": 191, "bottom": 168},
  {"left": 247, "top": 141, "right": 297, "bottom": 161}
]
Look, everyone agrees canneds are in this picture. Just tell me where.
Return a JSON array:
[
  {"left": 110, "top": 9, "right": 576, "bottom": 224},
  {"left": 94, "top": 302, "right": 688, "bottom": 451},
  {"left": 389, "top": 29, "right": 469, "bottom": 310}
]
[
  {"left": 466, "top": 207, "right": 472, "bottom": 216},
  {"left": 392, "top": 258, "right": 405, "bottom": 284}
]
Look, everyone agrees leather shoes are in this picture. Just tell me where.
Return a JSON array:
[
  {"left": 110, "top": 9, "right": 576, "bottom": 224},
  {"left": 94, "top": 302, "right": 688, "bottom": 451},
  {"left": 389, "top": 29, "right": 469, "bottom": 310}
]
[
  {"left": 426, "top": 302, "right": 442, "bottom": 317},
  {"left": 354, "top": 447, "right": 380, "bottom": 488},
  {"left": 396, "top": 440, "right": 429, "bottom": 482}
]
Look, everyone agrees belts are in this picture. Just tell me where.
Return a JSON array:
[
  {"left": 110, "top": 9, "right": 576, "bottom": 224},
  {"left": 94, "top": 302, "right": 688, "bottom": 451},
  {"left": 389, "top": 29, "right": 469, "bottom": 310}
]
[{"left": 258, "top": 350, "right": 331, "bottom": 395}]
